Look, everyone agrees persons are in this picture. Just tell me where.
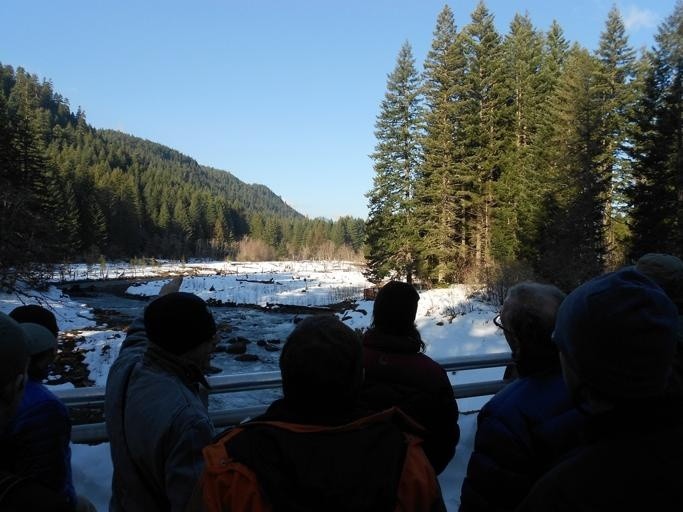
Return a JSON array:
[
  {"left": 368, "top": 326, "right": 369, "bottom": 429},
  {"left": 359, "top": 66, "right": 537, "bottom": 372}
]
[
  {"left": 180, "top": 312, "right": 448, "bottom": 510},
  {"left": 512, "top": 265, "right": 683, "bottom": 512},
  {"left": 356, "top": 280, "right": 461, "bottom": 479},
  {"left": 454, "top": 278, "right": 580, "bottom": 510},
  {"left": 635, "top": 252, "right": 683, "bottom": 314},
  {"left": 103, "top": 272, "right": 217, "bottom": 512},
  {"left": 8, "top": 305, "right": 74, "bottom": 496},
  {"left": 0, "top": 311, "right": 76, "bottom": 512}
]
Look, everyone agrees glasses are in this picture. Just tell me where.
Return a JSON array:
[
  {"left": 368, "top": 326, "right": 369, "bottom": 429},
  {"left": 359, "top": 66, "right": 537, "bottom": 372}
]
[{"left": 493, "top": 315, "right": 512, "bottom": 334}]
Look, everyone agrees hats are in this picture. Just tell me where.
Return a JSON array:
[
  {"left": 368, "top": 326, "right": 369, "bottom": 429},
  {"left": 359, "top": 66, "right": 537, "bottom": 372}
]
[
  {"left": 144, "top": 292, "right": 216, "bottom": 357},
  {"left": 551, "top": 267, "right": 679, "bottom": 395},
  {"left": 0, "top": 311, "right": 56, "bottom": 386},
  {"left": 637, "top": 253, "right": 683, "bottom": 299},
  {"left": 9, "top": 305, "right": 59, "bottom": 333}
]
